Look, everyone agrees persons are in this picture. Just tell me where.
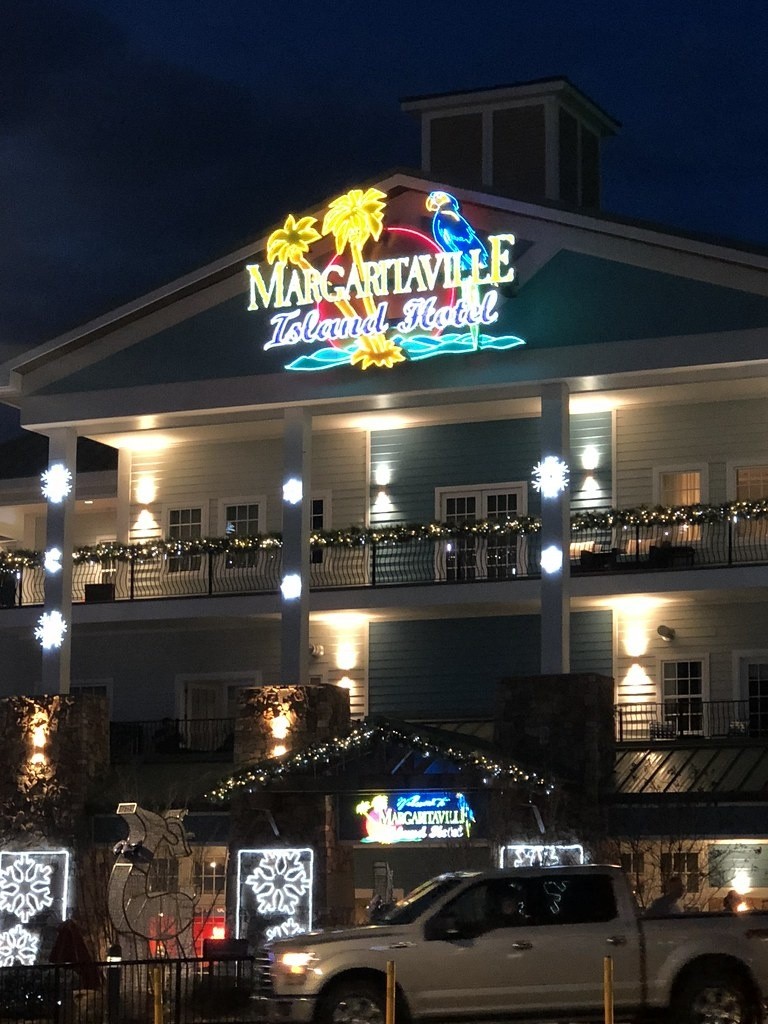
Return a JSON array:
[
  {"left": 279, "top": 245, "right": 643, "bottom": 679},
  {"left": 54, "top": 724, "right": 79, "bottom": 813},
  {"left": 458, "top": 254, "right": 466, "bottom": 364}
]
[
  {"left": 722, "top": 890, "right": 742, "bottom": 911},
  {"left": 644, "top": 877, "right": 684, "bottom": 915},
  {"left": 482, "top": 895, "right": 527, "bottom": 928}
]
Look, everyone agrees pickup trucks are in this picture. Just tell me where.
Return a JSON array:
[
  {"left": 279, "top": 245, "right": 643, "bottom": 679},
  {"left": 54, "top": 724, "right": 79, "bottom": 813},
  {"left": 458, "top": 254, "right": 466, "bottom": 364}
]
[{"left": 268, "top": 864, "right": 768, "bottom": 1024}]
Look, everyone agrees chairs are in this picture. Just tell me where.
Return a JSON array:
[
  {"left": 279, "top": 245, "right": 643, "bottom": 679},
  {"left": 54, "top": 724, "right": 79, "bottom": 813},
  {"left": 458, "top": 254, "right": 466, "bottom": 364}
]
[{"left": 570, "top": 537, "right": 661, "bottom": 573}]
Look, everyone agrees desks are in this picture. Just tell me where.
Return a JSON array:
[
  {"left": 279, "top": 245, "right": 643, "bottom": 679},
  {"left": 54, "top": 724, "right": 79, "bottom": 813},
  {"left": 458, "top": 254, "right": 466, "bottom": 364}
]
[{"left": 671, "top": 546, "right": 696, "bottom": 571}]
